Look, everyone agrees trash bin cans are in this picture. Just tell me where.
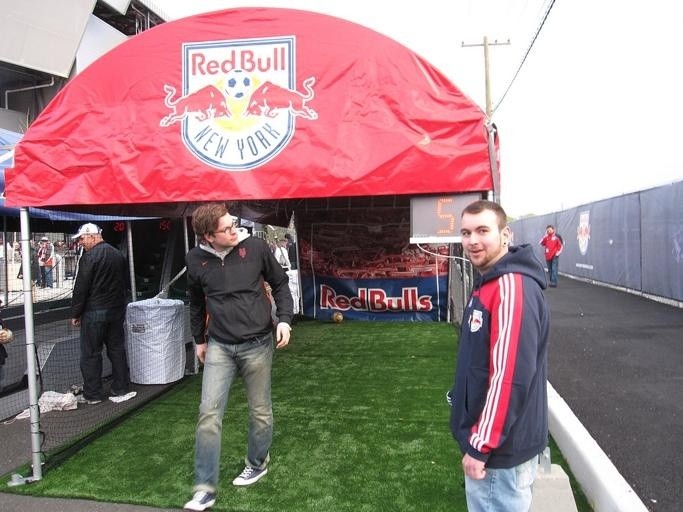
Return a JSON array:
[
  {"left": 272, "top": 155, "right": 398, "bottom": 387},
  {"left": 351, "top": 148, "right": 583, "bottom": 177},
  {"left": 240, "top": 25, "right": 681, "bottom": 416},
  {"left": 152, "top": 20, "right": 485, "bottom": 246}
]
[
  {"left": 126, "top": 297, "right": 186, "bottom": 385},
  {"left": 65, "top": 256, "right": 76, "bottom": 280}
]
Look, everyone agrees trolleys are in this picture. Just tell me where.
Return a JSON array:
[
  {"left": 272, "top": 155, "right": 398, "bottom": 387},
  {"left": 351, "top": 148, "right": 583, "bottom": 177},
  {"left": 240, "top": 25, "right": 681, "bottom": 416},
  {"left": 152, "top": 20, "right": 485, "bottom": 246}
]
[{"left": 64, "top": 255, "right": 76, "bottom": 281}]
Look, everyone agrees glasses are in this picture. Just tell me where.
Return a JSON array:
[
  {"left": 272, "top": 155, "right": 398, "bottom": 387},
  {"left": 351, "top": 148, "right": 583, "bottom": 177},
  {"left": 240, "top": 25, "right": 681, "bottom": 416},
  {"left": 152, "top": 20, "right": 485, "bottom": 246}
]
[{"left": 206, "top": 218, "right": 239, "bottom": 235}]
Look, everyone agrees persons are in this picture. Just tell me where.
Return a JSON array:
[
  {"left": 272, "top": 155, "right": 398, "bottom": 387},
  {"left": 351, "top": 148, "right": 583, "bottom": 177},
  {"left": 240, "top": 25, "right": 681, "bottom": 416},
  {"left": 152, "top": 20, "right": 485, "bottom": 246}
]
[
  {"left": 540, "top": 225, "right": 564, "bottom": 288},
  {"left": 16, "top": 237, "right": 67, "bottom": 289},
  {"left": 178, "top": 203, "right": 294, "bottom": 511},
  {"left": 1, "top": 298, "right": 15, "bottom": 367},
  {"left": 445, "top": 200, "right": 552, "bottom": 511},
  {"left": 69, "top": 223, "right": 129, "bottom": 404},
  {"left": 269, "top": 233, "right": 297, "bottom": 271}
]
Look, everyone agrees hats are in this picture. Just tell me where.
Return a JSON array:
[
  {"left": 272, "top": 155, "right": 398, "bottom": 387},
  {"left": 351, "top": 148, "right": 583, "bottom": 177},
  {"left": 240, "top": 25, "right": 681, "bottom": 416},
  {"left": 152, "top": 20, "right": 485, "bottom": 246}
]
[{"left": 70, "top": 222, "right": 103, "bottom": 239}]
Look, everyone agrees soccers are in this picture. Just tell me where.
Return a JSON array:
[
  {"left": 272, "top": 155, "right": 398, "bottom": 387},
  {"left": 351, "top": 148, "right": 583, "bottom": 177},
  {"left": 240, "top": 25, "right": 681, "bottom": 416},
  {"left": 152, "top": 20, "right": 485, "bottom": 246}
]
[
  {"left": 333, "top": 312, "right": 344, "bottom": 322},
  {"left": 1, "top": 329, "right": 14, "bottom": 344}
]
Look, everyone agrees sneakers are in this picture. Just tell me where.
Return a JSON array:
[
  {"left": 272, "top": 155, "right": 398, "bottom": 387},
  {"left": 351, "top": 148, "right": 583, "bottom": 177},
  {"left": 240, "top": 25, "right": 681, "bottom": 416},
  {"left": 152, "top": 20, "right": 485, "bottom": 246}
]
[
  {"left": 232, "top": 464, "right": 268, "bottom": 486},
  {"left": 71, "top": 393, "right": 102, "bottom": 405},
  {"left": 183, "top": 489, "right": 217, "bottom": 512},
  {"left": 548, "top": 282, "right": 558, "bottom": 289},
  {"left": 108, "top": 394, "right": 129, "bottom": 402}
]
